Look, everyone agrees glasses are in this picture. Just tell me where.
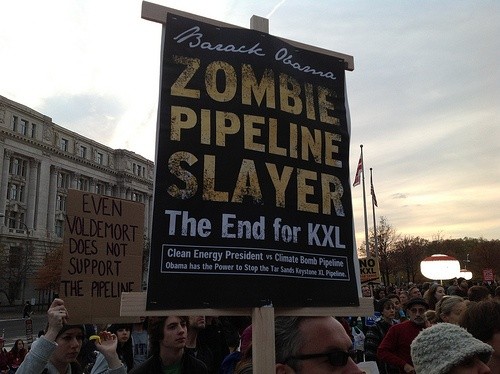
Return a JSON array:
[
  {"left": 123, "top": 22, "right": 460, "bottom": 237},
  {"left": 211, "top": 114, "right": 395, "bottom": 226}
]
[{"left": 282, "top": 349, "right": 363, "bottom": 366}]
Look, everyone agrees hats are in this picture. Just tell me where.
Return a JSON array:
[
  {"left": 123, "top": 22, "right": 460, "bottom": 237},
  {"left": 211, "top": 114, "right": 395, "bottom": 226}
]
[
  {"left": 406, "top": 298, "right": 429, "bottom": 309},
  {"left": 409, "top": 323, "right": 494, "bottom": 374},
  {"left": 46, "top": 321, "right": 85, "bottom": 342},
  {"left": 108, "top": 323, "right": 133, "bottom": 332}
]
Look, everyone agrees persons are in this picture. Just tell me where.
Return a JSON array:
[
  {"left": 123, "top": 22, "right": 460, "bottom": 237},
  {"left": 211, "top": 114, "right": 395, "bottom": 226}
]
[
  {"left": 276, "top": 276, "right": 500, "bottom": 374},
  {"left": 22, "top": 300, "right": 33, "bottom": 331},
  {"left": 0, "top": 315, "right": 253, "bottom": 374}
]
